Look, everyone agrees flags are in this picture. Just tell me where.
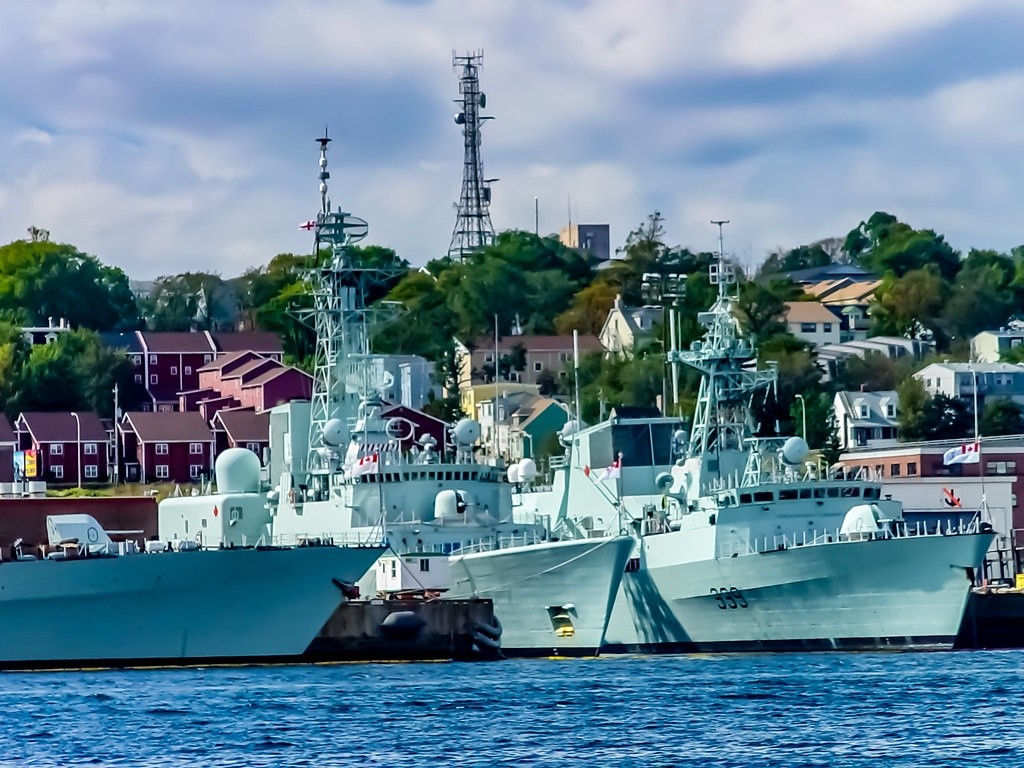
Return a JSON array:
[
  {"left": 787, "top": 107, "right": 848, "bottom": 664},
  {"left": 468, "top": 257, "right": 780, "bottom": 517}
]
[
  {"left": 298, "top": 220, "right": 316, "bottom": 231},
  {"left": 944, "top": 442, "right": 981, "bottom": 466},
  {"left": 596, "top": 457, "right": 620, "bottom": 482},
  {"left": 341, "top": 452, "right": 378, "bottom": 480}
]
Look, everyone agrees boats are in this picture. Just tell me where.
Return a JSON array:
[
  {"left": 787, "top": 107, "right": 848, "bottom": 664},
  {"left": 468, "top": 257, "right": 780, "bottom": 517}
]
[
  {"left": 510, "top": 416, "right": 694, "bottom": 536},
  {"left": 158, "top": 124, "right": 636, "bottom": 660},
  {"left": 604, "top": 220, "right": 999, "bottom": 653},
  {"left": 0, "top": 536, "right": 388, "bottom": 666}
]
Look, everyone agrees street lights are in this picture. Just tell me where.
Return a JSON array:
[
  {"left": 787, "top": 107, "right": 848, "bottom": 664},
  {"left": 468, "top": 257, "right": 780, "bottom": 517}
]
[
  {"left": 794, "top": 394, "right": 806, "bottom": 442},
  {"left": 71, "top": 412, "right": 81, "bottom": 489},
  {"left": 968, "top": 370, "right": 979, "bottom": 442}
]
